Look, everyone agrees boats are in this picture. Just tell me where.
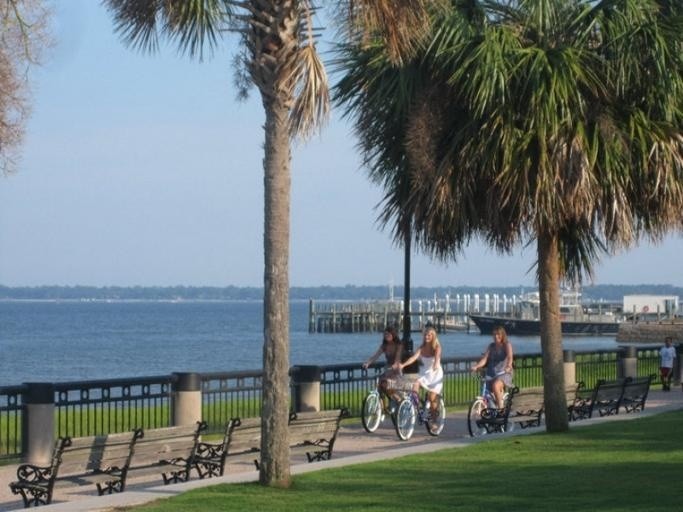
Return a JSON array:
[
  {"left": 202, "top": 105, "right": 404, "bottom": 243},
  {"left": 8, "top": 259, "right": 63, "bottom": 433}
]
[{"left": 426, "top": 309, "right": 469, "bottom": 330}]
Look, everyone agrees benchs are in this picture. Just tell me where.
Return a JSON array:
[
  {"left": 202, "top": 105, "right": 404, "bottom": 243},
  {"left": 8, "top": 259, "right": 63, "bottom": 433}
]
[
  {"left": 476, "top": 373, "right": 657, "bottom": 434},
  {"left": 187, "top": 408, "right": 350, "bottom": 480},
  {"left": 8, "top": 420, "right": 208, "bottom": 508}
]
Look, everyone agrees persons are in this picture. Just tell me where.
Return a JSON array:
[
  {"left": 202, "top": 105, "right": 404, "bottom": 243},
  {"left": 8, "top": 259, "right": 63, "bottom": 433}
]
[
  {"left": 658, "top": 336, "right": 677, "bottom": 390},
  {"left": 391, "top": 324, "right": 444, "bottom": 429},
  {"left": 362, "top": 327, "right": 405, "bottom": 404},
  {"left": 470, "top": 325, "right": 513, "bottom": 416}
]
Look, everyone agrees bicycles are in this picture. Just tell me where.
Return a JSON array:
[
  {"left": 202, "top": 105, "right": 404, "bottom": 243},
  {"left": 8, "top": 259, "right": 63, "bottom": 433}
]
[
  {"left": 362, "top": 366, "right": 409, "bottom": 433},
  {"left": 395, "top": 364, "right": 447, "bottom": 441},
  {"left": 468, "top": 367, "right": 517, "bottom": 437}
]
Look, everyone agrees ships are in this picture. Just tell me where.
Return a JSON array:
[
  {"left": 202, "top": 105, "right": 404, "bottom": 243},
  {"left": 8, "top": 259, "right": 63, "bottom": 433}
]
[{"left": 466, "top": 289, "right": 636, "bottom": 335}]
